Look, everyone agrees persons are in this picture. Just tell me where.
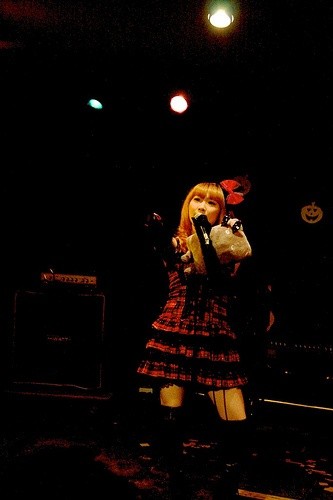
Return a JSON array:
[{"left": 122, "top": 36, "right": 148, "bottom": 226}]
[{"left": 144, "top": 181, "right": 264, "bottom": 500}]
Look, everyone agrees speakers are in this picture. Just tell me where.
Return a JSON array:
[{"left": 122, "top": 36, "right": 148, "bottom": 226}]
[{"left": 12, "top": 279, "right": 108, "bottom": 392}]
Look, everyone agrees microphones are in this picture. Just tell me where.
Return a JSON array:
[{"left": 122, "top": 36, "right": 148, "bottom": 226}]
[{"left": 194, "top": 212, "right": 209, "bottom": 245}]
